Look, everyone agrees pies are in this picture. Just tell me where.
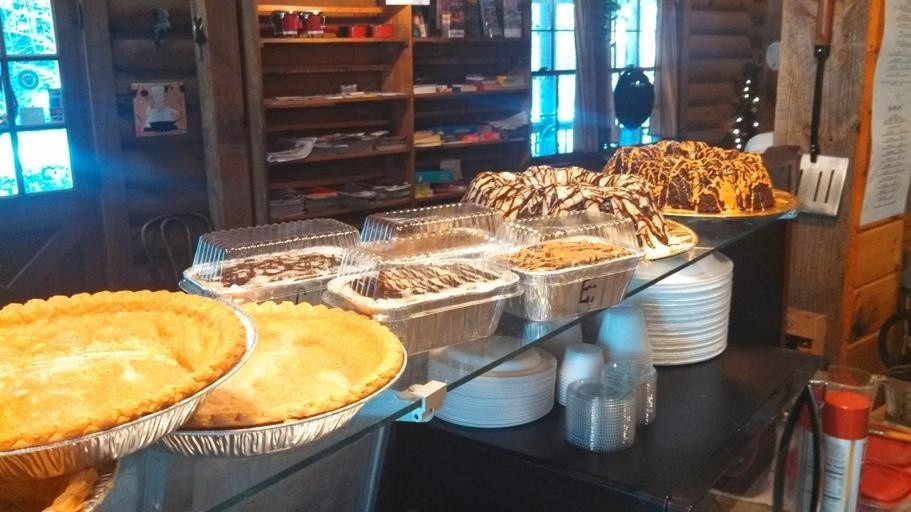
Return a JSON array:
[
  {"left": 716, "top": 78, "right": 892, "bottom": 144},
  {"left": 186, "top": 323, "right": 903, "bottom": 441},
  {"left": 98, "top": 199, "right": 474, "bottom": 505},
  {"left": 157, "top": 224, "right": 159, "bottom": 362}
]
[{"left": 178, "top": 295, "right": 408, "bottom": 458}]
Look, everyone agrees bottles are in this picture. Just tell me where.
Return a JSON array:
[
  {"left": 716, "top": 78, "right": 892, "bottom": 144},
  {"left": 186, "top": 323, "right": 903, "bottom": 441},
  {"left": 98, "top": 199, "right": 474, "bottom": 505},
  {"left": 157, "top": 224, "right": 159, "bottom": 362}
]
[{"left": 796, "top": 367, "right": 869, "bottom": 512}]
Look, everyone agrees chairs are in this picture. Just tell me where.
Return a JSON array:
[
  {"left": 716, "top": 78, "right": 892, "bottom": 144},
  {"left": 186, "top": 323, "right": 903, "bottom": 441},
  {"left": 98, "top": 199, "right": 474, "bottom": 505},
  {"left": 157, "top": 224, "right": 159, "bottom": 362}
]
[{"left": 140, "top": 213, "right": 211, "bottom": 294}]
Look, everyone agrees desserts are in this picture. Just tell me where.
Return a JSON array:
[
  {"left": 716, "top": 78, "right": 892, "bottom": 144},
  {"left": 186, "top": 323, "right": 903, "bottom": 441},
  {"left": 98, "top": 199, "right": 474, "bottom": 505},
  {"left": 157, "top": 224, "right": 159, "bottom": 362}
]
[{"left": 190, "top": 137, "right": 799, "bottom": 314}]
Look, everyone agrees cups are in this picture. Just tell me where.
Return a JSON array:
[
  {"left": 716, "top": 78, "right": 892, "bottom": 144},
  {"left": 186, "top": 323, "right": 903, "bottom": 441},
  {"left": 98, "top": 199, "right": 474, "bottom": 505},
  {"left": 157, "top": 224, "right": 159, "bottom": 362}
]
[
  {"left": 884, "top": 364, "right": 911, "bottom": 429},
  {"left": 269, "top": 9, "right": 393, "bottom": 38}
]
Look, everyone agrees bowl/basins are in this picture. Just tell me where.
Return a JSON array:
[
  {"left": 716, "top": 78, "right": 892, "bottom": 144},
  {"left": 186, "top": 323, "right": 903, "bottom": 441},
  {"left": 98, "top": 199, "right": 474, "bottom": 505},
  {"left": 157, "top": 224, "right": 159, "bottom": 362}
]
[
  {"left": 613, "top": 68, "right": 653, "bottom": 129},
  {"left": 544, "top": 306, "right": 654, "bottom": 408}
]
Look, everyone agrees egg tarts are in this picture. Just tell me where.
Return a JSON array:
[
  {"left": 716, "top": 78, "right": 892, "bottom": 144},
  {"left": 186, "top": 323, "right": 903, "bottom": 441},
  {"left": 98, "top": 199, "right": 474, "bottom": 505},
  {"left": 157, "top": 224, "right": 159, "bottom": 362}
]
[
  {"left": 0, "top": 290, "right": 247, "bottom": 452},
  {"left": 0, "top": 467, "right": 100, "bottom": 512}
]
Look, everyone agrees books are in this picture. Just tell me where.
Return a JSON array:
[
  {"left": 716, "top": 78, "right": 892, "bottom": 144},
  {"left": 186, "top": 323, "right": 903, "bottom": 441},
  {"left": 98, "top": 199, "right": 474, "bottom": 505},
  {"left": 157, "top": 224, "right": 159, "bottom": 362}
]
[
  {"left": 267, "top": 176, "right": 411, "bottom": 218},
  {"left": 268, "top": 128, "right": 408, "bottom": 162},
  {"left": 414, "top": 130, "right": 501, "bottom": 147},
  {"left": 413, "top": 73, "right": 526, "bottom": 94},
  {"left": 414, "top": 166, "right": 468, "bottom": 199},
  {"left": 415, "top": 1, "right": 522, "bottom": 39}
]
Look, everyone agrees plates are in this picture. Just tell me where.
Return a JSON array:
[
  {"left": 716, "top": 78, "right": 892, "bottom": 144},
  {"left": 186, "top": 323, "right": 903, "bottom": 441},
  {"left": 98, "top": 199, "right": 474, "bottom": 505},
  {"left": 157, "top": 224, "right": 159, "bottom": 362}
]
[
  {"left": 585, "top": 251, "right": 737, "bottom": 365},
  {"left": 428, "top": 337, "right": 558, "bottom": 430}
]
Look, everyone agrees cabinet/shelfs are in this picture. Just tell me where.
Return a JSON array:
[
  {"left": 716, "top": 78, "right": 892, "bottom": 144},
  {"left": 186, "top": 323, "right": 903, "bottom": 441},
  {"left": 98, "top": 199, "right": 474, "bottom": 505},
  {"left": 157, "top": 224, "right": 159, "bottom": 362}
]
[
  {"left": 211, "top": 226, "right": 829, "bottom": 512},
  {"left": 238, "top": 0, "right": 534, "bottom": 225},
  {"left": 655, "top": 2, "right": 779, "bottom": 146}
]
